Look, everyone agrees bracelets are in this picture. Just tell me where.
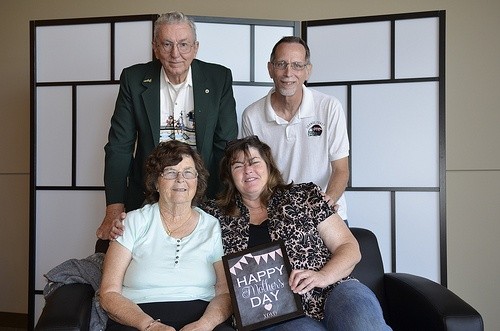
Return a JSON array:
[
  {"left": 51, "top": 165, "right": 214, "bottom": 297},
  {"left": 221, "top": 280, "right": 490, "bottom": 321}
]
[{"left": 144, "top": 318, "right": 160, "bottom": 331}]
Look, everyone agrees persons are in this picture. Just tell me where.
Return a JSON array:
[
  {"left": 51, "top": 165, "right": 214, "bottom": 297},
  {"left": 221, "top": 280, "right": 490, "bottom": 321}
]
[
  {"left": 99, "top": 140, "right": 232, "bottom": 331},
  {"left": 109, "top": 136, "right": 393, "bottom": 331},
  {"left": 95, "top": 12, "right": 239, "bottom": 239},
  {"left": 242, "top": 36, "right": 350, "bottom": 229}
]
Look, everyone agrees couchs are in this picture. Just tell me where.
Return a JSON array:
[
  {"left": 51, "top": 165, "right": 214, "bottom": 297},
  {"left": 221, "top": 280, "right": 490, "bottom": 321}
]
[{"left": 33, "top": 227, "right": 484, "bottom": 331}]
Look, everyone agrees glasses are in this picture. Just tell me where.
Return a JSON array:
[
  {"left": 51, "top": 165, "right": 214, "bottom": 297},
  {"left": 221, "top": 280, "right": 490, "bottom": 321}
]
[
  {"left": 270, "top": 59, "right": 309, "bottom": 71},
  {"left": 159, "top": 167, "right": 199, "bottom": 179},
  {"left": 152, "top": 38, "right": 195, "bottom": 53}
]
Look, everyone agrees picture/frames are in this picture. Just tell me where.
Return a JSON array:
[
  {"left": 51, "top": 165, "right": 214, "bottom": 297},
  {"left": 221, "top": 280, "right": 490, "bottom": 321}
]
[{"left": 222, "top": 238, "right": 306, "bottom": 331}]
